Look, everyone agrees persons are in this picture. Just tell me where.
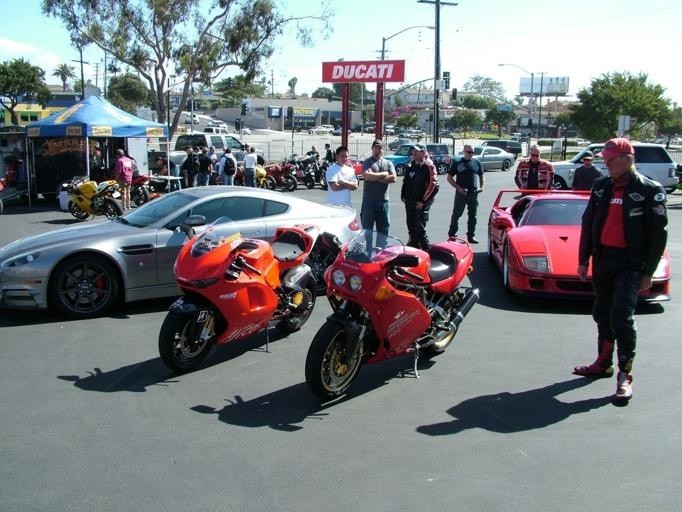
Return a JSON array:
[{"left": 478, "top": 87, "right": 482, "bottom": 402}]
[
  {"left": 359, "top": 138, "right": 397, "bottom": 257},
  {"left": 571, "top": 151, "right": 602, "bottom": 192},
  {"left": 514, "top": 144, "right": 554, "bottom": 194},
  {"left": 114, "top": 149, "right": 133, "bottom": 212},
  {"left": 125, "top": 155, "right": 139, "bottom": 178},
  {"left": 574, "top": 136, "right": 669, "bottom": 401},
  {"left": 185, "top": 113, "right": 200, "bottom": 125},
  {"left": 322, "top": 142, "right": 335, "bottom": 160},
  {"left": 446, "top": 144, "right": 485, "bottom": 243},
  {"left": 400, "top": 142, "right": 439, "bottom": 253},
  {"left": 325, "top": 146, "right": 359, "bottom": 206},
  {"left": 154, "top": 145, "right": 268, "bottom": 192},
  {"left": 306, "top": 145, "right": 320, "bottom": 160}
]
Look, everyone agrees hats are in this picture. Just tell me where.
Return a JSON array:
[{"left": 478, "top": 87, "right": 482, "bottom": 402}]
[
  {"left": 595, "top": 137, "right": 634, "bottom": 158},
  {"left": 582, "top": 151, "right": 593, "bottom": 161},
  {"left": 409, "top": 143, "right": 428, "bottom": 152},
  {"left": 372, "top": 139, "right": 385, "bottom": 148}
]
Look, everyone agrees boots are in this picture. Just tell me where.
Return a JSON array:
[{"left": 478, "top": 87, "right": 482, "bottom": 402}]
[
  {"left": 571, "top": 334, "right": 615, "bottom": 378},
  {"left": 613, "top": 346, "right": 636, "bottom": 402}
]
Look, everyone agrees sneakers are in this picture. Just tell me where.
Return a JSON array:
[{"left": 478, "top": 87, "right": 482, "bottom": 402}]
[{"left": 467, "top": 237, "right": 480, "bottom": 244}]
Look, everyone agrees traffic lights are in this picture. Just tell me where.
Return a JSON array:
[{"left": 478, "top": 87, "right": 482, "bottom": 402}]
[
  {"left": 241, "top": 103, "right": 247, "bottom": 116},
  {"left": 193, "top": 101, "right": 198, "bottom": 110},
  {"left": 234, "top": 118, "right": 240, "bottom": 131}
]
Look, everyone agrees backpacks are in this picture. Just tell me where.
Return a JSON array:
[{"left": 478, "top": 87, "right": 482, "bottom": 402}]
[{"left": 221, "top": 155, "right": 236, "bottom": 176}]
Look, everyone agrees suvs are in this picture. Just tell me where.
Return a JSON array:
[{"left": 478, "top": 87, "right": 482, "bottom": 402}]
[
  {"left": 381, "top": 144, "right": 453, "bottom": 177},
  {"left": 204, "top": 124, "right": 241, "bottom": 140},
  {"left": 186, "top": 113, "right": 200, "bottom": 125},
  {"left": 551, "top": 140, "right": 681, "bottom": 191}
]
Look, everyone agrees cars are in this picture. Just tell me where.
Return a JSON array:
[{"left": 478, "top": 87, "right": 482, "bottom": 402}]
[
  {"left": 207, "top": 122, "right": 215, "bottom": 127},
  {"left": 347, "top": 156, "right": 366, "bottom": 179},
  {"left": 574, "top": 138, "right": 590, "bottom": 146},
  {"left": 454, "top": 133, "right": 524, "bottom": 172},
  {"left": 438, "top": 130, "right": 448, "bottom": 135},
  {"left": 306, "top": 123, "right": 426, "bottom": 142},
  {"left": 212, "top": 120, "right": 227, "bottom": 130}
]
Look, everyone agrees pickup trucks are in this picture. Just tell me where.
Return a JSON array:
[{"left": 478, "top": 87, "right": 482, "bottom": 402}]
[{"left": 148, "top": 133, "right": 265, "bottom": 177}]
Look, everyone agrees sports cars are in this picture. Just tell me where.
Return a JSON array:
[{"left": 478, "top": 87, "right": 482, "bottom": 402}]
[
  {"left": 0, "top": 185, "right": 359, "bottom": 315},
  {"left": 486, "top": 189, "right": 673, "bottom": 305}
]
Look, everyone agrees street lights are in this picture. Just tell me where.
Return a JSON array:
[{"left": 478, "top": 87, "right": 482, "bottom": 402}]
[
  {"left": 497, "top": 63, "right": 534, "bottom": 137},
  {"left": 377, "top": 24, "right": 435, "bottom": 139}
]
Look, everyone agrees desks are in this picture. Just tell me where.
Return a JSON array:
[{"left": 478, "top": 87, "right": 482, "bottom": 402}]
[{"left": 150, "top": 175, "right": 184, "bottom": 193}]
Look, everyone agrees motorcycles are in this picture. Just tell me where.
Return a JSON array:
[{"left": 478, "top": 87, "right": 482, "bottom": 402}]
[
  {"left": 206, "top": 152, "right": 332, "bottom": 191},
  {"left": 305, "top": 228, "right": 484, "bottom": 398},
  {"left": 131, "top": 174, "right": 155, "bottom": 207},
  {"left": 157, "top": 214, "right": 317, "bottom": 370},
  {"left": 63, "top": 175, "right": 125, "bottom": 220}
]
